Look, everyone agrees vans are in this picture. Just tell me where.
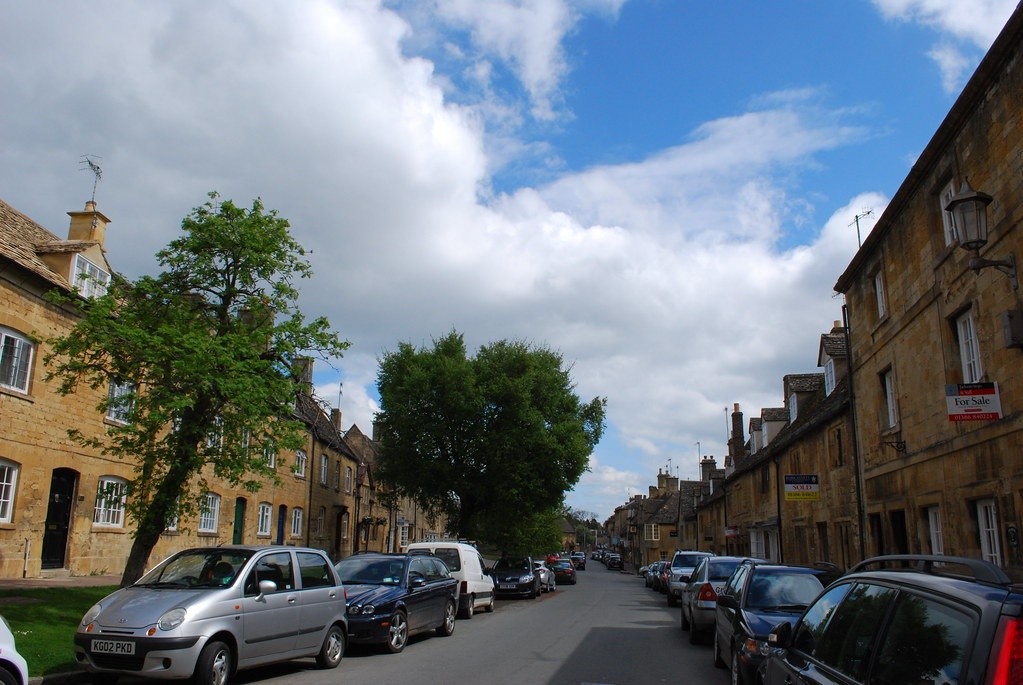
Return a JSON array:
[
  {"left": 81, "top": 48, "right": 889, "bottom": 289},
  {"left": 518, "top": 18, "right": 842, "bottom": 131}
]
[{"left": 406, "top": 542, "right": 495, "bottom": 619}]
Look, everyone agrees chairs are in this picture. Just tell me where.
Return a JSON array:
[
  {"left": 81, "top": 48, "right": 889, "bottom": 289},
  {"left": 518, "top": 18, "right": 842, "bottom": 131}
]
[
  {"left": 748, "top": 578, "right": 771, "bottom": 605},
  {"left": 213, "top": 562, "right": 235, "bottom": 577},
  {"left": 257, "top": 563, "right": 283, "bottom": 582}
]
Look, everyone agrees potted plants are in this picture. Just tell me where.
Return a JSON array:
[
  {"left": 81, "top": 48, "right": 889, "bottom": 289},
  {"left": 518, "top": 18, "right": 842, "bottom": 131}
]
[
  {"left": 363, "top": 512, "right": 376, "bottom": 525},
  {"left": 376, "top": 514, "right": 387, "bottom": 526}
]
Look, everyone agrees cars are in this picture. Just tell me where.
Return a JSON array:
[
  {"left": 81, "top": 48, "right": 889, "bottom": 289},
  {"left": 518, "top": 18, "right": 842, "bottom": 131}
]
[
  {"left": 550, "top": 559, "right": 576, "bottom": 585},
  {"left": 534, "top": 560, "right": 557, "bottom": 593},
  {"left": 646, "top": 563, "right": 658, "bottom": 588},
  {"left": 488, "top": 556, "right": 541, "bottom": 600},
  {"left": 544, "top": 553, "right": 560, "bottom": 564},
  {"left": 0, "top": 614, "right": 29, "bottom": 685},
  {"left": 652, "top": 561, "right": 666, "bottom": 591},
  {"left": 679, "top": 556, "right": 775, "bottom": 646},
  {"left": 590, "top": 549, "right": 624, "bottom": 570},
  {"left": 74, "top": 546, "right": 348, "bottom": 685},
  {"left": 639, "top": 562, "right": 658, "bottom": 578},
  {"left": 574, "top": 551, "right": 587, "bottom": 563},
  {"left": 570, "top": 556, "right": 585, "bottom": 570},
  {"left": 659, "top": 562, "right": 671, "bottom": 595}
]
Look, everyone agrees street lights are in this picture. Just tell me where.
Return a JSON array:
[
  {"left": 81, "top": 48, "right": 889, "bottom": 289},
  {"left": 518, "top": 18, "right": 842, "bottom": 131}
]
[{"left": 353, "top": 462, "right": 367, "bottom": 555}]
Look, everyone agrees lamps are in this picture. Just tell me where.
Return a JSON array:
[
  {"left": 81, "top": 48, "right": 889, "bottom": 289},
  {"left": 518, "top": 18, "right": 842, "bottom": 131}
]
[{"left": 944, "top": 175, "right": 1018, "bottom": 290}]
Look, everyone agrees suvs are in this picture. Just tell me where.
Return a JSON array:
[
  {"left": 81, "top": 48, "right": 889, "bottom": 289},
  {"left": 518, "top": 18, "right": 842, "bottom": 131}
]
[
  {"left": 752, "top": 554, "right": 1023, "bottom": 685},
  {"left": 713, "top": 559, "right": 844, "bottom": 685},
  {"left": 666, "top": 548, "right": 718, "bottom": 607},
  {"left": 322, "top": 550, "right": 459, "bottom": 653}
]
